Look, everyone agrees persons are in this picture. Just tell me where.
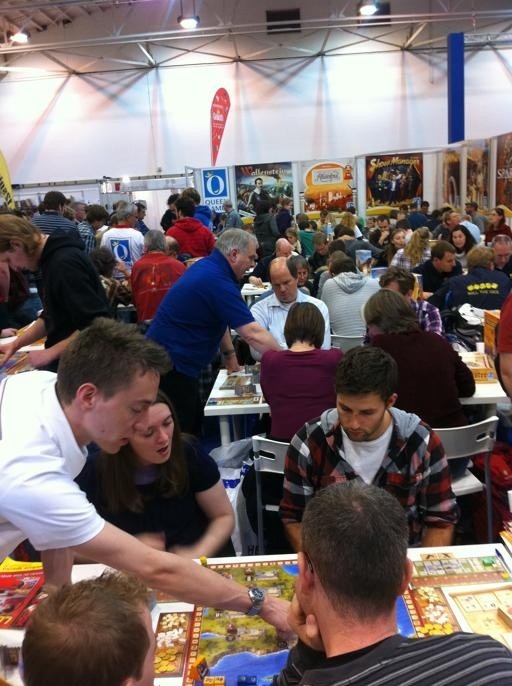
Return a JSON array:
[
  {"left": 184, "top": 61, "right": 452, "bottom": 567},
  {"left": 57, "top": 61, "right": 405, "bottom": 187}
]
[
  {"left": 0, "top": 317, "right": 298, "bottom": 642},
  {"left": 24, "top": 572, "right": 155, "bottom": 684},
  {"left": 280, "top": 348, "right": 458, "bottom": 550},
  {"left": 269, "top": 481, "right": 512, "bottom": 684},
  {"left": 238, "top": 177, "right": 292, "bottom": 216},
  {"left": 77, "top": 392, "right": 237, "bottom": 557},
  {"left": 377, "top": 171, "right": 413, "bottom": 202},
  {"left": 0, "top": 187, "right": 511, "bottom": 480}
]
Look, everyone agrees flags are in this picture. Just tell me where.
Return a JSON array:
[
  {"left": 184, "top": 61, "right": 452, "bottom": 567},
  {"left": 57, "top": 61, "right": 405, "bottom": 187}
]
[{"left": 0, "top": 151, "right": 16, "bottom": 209}]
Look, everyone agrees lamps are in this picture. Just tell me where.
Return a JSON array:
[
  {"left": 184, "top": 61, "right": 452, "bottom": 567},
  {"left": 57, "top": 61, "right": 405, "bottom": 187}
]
[
  {"left": 5, "top": 11, "right": 35, "bottom": 45},
  {"left": 356, "top": 1, "right": 384, "bottom": 18},
  {"left": 178, "top": 2, "right": 201, "bottom": 31}
]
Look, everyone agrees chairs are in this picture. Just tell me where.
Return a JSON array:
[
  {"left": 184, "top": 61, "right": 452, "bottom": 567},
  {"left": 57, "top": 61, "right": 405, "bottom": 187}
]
[
  {"left": 429, "top": 414, "right": 501, "bottom": 543},
  {"left": 325, "top": 333, "right": 366, "bottom": 352},
  {"left": 247, "top": 433, "right": 301, "bottom": 557}
]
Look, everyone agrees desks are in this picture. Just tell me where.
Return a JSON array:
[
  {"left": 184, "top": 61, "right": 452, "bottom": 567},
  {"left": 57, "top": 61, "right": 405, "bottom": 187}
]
[
  {"left": 1, "top": 536, "right": 511, "bottom": 686},
  {"left": 240, "top": 281, "right": 273, "bottom": 307},
  {"left": 205, "top": 347, "right": 510, "bottom": 455},
  {"left": 1, "top": 328, "right": 52, "bottom": 379}
]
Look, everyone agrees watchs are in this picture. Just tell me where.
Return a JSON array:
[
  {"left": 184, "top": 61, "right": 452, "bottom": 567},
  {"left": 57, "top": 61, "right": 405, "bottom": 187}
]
[{"left": 248, "top": 589, "right": 265, "bottom": 615}]
